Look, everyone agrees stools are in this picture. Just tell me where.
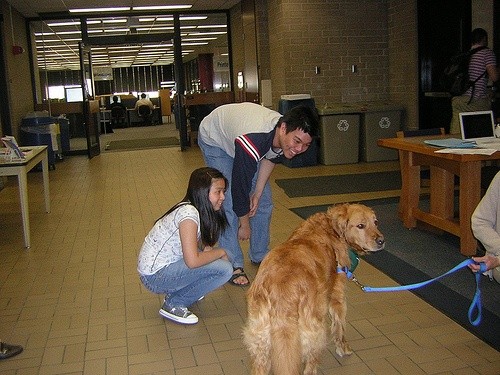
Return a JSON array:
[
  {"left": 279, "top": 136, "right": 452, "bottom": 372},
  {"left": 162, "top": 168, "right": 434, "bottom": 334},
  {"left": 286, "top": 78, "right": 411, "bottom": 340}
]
[{"left": 162, "top": 113, "right": 172, "bottom": 124}]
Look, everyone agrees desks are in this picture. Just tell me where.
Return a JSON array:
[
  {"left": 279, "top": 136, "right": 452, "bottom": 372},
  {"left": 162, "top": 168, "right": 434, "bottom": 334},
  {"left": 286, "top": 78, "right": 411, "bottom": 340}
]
[
  {"left": 378, "top": 134, "right": 500, "bottom": 255},
  {"left": 100, "top": 107, "right": 161, "bottom": 126},
  {"left": 0, "top": 145, "right": 50, "bottom": 248}
]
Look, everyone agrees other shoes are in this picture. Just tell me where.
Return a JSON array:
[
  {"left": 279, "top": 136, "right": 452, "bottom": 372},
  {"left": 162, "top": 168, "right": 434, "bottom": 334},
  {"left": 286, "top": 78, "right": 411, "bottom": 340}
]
[{"left": 0, "top": 342, "right": 23, "bottom": 359}]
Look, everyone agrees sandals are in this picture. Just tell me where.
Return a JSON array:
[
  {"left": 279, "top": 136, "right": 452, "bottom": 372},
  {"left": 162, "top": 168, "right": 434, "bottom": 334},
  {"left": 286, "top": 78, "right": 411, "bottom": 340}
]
[{"left": 228, "top": 266, "right": 250, "bottom": 286}]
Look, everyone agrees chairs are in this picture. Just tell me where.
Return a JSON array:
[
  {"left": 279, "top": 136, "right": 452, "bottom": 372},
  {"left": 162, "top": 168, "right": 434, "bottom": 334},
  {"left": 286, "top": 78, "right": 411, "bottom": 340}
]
[
  {"left": 110, "top": 106, "right": 126, "bottom": 127},
  {"left": 397, "top": 128, "right": 459, "bottom": 222},
  {"left": 138, "top": 105, "right": 153, "bottom": 126}
]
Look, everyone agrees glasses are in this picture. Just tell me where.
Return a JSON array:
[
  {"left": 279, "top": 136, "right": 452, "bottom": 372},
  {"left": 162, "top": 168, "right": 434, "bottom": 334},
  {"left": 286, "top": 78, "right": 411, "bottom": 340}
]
[{"left": 461, "top": 141, "right": 476, "bottom": 145}]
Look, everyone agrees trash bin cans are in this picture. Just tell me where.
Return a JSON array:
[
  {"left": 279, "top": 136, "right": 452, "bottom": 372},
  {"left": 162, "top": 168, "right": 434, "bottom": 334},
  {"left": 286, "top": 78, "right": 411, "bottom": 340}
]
[
  {"left": 55, "top": 117, "right": 71, "bottom": 152},
  {"left": 316, "top": 105, "right": 362, "bottom": 164},
  {"left": 361, "top": 106, "right": 404, "bottom": 162},
  {"left": 18, "top": 116, "right": 57, "bottom": 174},
  {"left": 279, "top": 94, "right": 319, "bottom": 168}
]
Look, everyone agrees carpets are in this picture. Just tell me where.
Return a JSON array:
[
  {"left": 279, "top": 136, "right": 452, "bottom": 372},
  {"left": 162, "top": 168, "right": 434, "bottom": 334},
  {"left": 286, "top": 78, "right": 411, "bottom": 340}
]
[
  {"left": 275, "top": 167, "right": 460, "bottom": 198},
  {"left": 289, "top": 190, "right": 500, "bottom": 352}
]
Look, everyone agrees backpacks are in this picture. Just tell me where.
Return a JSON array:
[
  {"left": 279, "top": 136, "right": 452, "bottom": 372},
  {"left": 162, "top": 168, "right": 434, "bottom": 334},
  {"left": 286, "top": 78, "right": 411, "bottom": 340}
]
[{"left": 444, "top": 46, "right": 488, "bottom": 96}]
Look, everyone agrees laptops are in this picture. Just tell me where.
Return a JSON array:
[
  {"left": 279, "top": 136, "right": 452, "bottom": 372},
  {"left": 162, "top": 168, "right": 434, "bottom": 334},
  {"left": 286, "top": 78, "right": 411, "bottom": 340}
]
[{"left": 459, "top": 111, "right": 500, "bottom": 146}]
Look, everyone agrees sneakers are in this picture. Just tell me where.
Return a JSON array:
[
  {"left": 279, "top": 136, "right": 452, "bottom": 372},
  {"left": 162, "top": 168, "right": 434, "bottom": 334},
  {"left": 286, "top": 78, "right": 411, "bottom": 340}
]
[{"left": 159, "top": 306, "right": 199, "bottom": 324}]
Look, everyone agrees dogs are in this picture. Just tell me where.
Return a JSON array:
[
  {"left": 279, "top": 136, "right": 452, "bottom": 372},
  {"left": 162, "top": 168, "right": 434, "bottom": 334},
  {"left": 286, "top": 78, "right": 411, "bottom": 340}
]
[{"left": 237, "top": 203, "right": 386, "bottom": 375}]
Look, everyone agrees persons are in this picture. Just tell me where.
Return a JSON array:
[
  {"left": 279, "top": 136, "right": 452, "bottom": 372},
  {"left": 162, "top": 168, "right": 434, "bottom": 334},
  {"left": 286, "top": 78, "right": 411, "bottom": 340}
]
[
  {"left": 110, "top": 96, "right": 126, "bottom": 111},
  {"left": 135, "top": 93, "right": 154, "bottom": 116},
  {"left": 468, "top": 172, "right": 500, "bottom": 285},
  {"left": 137, "top": 168, "right": 234, "bottom": 324},
  {"left": 450, "top": 28, "right": 500, "bottom": 134},
  {"left": 198, "top": 102, "right": 317, "bottom": 288}
]
